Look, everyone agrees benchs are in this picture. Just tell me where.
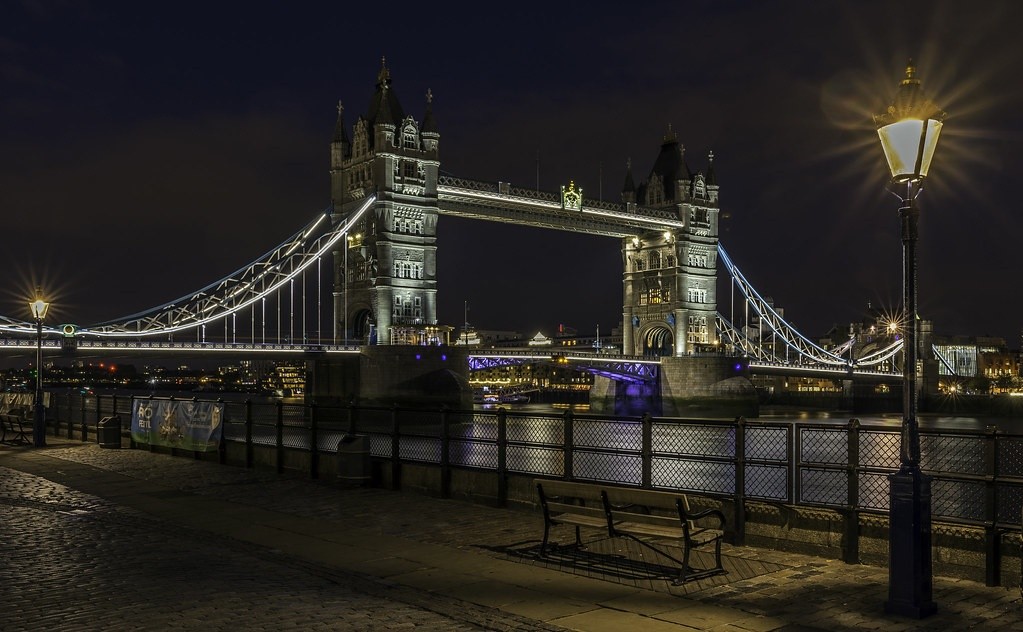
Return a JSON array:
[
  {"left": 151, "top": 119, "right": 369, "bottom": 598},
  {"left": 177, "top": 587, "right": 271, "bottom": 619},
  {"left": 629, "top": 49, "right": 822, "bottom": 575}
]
[
  {"left": 0, "top": 414, "right": 32, "bottom": 447},
  {"left": 533, "top": 479, "right": 729, "bottom": 586}
]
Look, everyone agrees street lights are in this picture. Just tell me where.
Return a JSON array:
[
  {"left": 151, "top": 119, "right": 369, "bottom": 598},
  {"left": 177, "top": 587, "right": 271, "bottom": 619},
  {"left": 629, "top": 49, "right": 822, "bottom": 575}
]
[
  {"left": 870, "top": 50, "right": 950, "bottom": 619},
  {"left": 28, "top": 285, "right": 50, "bottom": 447}
]
